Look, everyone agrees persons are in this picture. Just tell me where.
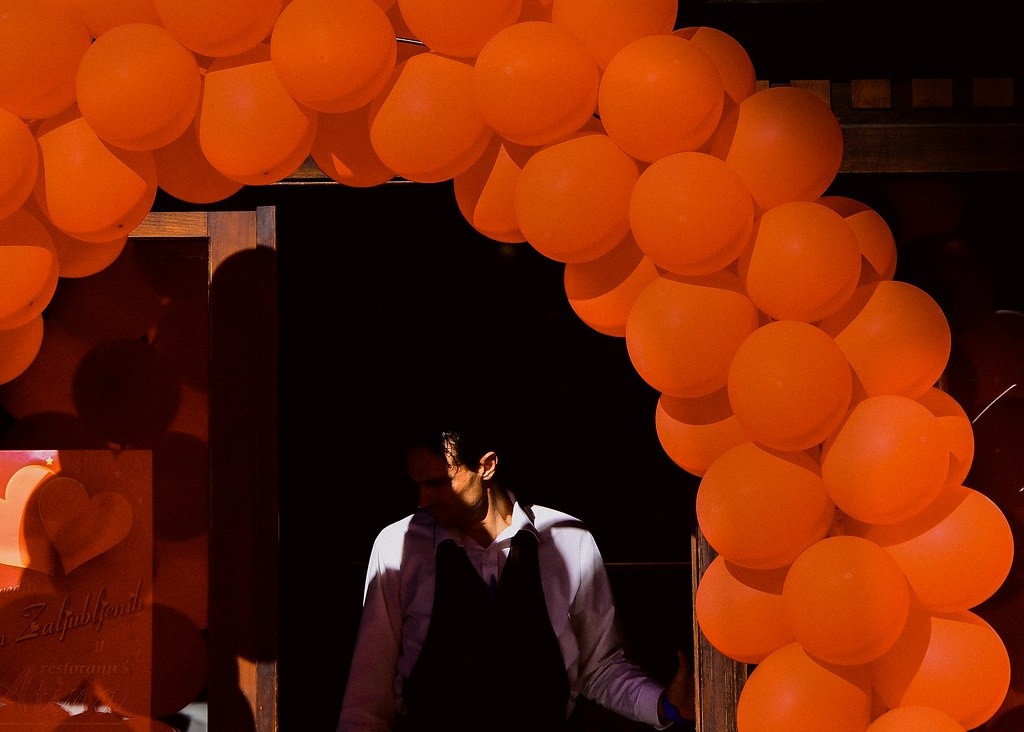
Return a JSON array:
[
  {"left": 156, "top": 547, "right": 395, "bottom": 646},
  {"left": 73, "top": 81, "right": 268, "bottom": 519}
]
[{"left": 337, "top": 419, "right": 695, "bottom": 732}]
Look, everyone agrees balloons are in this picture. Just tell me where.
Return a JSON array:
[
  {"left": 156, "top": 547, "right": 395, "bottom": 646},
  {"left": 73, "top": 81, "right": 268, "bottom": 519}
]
[{"left": 0, "top": 0, "right": 1016, "bottom": 732}]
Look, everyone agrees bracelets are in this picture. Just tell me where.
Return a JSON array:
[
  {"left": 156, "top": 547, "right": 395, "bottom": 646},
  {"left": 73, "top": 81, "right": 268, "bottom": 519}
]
[{"left": 663, "top": 694, "right": 686, "bottom": 724}]
